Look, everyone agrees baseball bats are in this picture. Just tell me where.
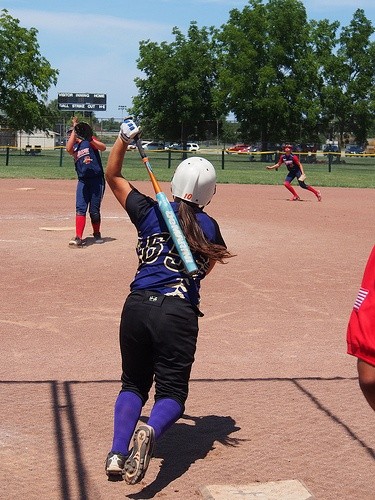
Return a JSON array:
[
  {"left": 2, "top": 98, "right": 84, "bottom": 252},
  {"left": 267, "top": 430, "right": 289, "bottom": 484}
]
[{"left": 124, "top": 117, "right": 199, "bottom": 275}]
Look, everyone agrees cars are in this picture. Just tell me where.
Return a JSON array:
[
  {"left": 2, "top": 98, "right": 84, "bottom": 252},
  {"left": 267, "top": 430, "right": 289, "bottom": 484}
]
[
  {"left": 225, "top": 144, "right": 262, "bottom": 154},
  {"left": 125, "top": 140, "right": 165, "bottom": 151},
  {"left": 345, "top": 144, "right": 363, "bottom": 154},
  {"left": 282, "top": 142, "right": 318, "bottom": 153},
  {"left": 168, "top": 143, "right": 199, "bottom": 151},
  {"left": 324, "top": 145, "right": 339, "bottom": 152}
]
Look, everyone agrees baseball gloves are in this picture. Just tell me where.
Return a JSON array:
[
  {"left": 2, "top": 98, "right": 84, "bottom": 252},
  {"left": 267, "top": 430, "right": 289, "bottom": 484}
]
[
  {"left": 296, "top": 171, "right": 307, "bottom": 182},
  {"left": 74, "top": 122, "right": 93, "bottom": 142}
]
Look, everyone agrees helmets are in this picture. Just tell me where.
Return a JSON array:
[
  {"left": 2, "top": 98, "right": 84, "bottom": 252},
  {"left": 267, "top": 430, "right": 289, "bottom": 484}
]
[
  {"left": 170, "top": 156, "right": 216, "bottom": 206},
  {"left": 284, "top": 144, "right": 292, "bottom": 151}
]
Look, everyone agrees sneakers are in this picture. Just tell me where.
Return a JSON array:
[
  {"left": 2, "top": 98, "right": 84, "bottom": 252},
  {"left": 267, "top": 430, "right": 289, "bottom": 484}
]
[
  {"left": 122, "top": 424, "right": 155, "bottom": 485},
  {"left": 104, "top": 452, "right": 129, "bottom": 479}
]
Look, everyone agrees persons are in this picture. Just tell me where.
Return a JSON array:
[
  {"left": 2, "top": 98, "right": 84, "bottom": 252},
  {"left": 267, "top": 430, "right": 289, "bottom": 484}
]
[
  {"left": 66, "top": 117, "right": 106, "bottom": 245},
  {"left": 345, "top": 244, "right": 375, "bottom": 411},
  {"left": 103, "top": 121, "right": 226, "bottom": 485},
  {"left": 266, "top": 144, "right": 321, "bottom": 201}
]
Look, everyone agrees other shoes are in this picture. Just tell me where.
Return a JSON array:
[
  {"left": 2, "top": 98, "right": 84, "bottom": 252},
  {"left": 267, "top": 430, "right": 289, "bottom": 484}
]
[
  {"left": 316, "top": 190, "right": 321, "bottom": 201},
  {"left": 69, "top": 237, "right": 83, "bottom": 247},
  {"left": 290, "top": 197, "right": 300, "bottom": 201},
  {"left": 93, "top": 232, "right": 103, "bottom": 243}
]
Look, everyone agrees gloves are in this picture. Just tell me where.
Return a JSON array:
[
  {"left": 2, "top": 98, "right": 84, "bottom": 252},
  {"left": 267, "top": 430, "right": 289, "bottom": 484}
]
[{"left": 118, "top": 119, "right": 139, "bottom": 144}]
